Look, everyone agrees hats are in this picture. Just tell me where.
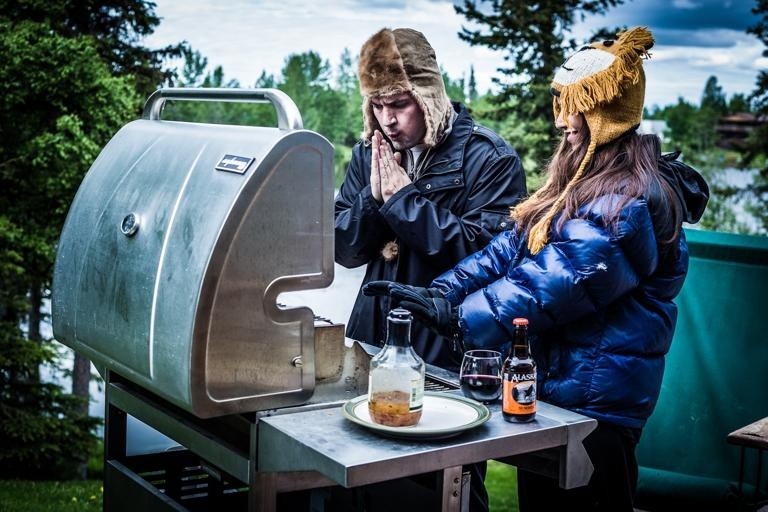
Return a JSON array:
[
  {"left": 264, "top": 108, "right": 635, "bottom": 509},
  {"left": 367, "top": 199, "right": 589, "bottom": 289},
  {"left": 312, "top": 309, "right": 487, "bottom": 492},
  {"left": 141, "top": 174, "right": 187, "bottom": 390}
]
[
  {"left": 355, "top": 26, "right": 455, "bottom": 150},
  {"left": 548, "top": 25, "right": 657, "bottom": 146}
]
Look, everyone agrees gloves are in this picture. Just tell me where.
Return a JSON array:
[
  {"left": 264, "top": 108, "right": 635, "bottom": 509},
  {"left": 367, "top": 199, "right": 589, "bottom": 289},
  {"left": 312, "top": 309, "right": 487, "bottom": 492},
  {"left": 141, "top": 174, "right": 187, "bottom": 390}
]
[{"left": 358, "top": 279, "right": 459, "bottom": 338}]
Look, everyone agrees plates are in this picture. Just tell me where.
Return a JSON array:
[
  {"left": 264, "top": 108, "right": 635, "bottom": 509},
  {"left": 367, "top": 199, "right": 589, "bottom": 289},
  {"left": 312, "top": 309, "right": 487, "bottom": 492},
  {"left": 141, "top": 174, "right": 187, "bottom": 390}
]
[{"left": 345, "top": 391, "right": 501, "bottom": 442}]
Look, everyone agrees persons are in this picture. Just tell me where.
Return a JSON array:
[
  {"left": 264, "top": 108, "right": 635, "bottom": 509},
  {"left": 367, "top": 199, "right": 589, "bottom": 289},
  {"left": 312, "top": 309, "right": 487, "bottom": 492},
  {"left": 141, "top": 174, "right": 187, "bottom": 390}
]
[
  {"left": 333, "top": 29, "right": 528, "bottom": 512},
  {"left": 360, "top": 25, "right": 709, "bottom": 511}
]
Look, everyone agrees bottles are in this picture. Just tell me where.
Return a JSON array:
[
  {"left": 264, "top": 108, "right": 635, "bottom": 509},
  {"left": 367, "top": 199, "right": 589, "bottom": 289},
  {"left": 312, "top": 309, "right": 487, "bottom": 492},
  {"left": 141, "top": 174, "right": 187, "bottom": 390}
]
[
  {"left": 369, "top": 308, "right": 424, "bottom": 424},
  {"left": 504, "top": 317, "right": 536, "bottom": 426},
  {"left": 461, "top": 349, "right": 504, "bottom": 403}
]
